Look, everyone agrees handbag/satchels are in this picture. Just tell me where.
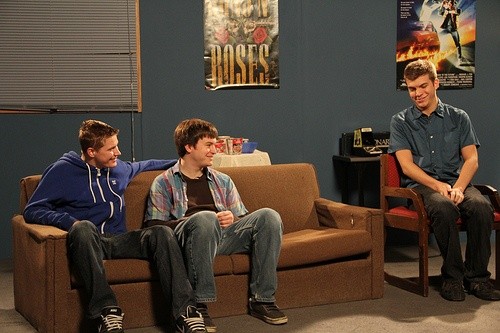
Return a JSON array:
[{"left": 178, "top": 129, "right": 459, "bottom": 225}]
[{"left": 339, "top": 128, "right": 391, "bottom": 157}]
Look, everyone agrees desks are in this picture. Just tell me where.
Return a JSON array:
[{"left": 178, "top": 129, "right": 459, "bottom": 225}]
[
  {"left": 208, "top": 149, "right": 272, "bottom": 168},
  {"left": 333, "top": 154, "right": 378, "bottom": 209}
]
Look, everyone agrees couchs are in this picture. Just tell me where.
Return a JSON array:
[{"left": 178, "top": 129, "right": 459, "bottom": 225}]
[{"left": 12, "top": 163, "right": 384, "bottom": 333}]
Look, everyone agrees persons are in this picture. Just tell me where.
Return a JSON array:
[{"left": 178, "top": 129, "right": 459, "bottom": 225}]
[
  {"left": 143, "top": 120, "right": 289, "bottom": 333},
  {"left": 388, "top": 59, "right": 500, "bottom": 301},
  {"left": 439, "top": 0, "right": 463, "bottom": 62},
  {"left": 24, "top": 120, "right": 208, "bottom": 333}
]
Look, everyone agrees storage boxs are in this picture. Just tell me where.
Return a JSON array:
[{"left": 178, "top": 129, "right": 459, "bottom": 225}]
[
  {"left": 342, "top": 129, "right": 390, "bottom": 157},
  {"left": 215, "top": 136, "right": 242, "bottom": 154}
]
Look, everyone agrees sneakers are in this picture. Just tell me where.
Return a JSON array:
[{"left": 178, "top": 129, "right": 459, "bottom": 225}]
[
  {"left": 250, "top": 303, "right": 288, "bottom": 324},
  {"left": 170, "top": 307, "right": 206, "bottom": 333},
  {"left": 195, "top": 305, "right": 216, "bottom": 333},
  {"left": 440, "top": 278, "right": 466, "bottom": 302},
  {"left": 463, "top": 280, "right": 500, "bottom": 302},
  {"left": 97, "top": 306, "right": 125, "bottom": 333}
]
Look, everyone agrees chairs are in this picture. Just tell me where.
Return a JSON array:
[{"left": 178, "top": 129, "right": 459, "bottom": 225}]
[{"left": 380, "top": 154, "right": 500, "bottom": 297}]
